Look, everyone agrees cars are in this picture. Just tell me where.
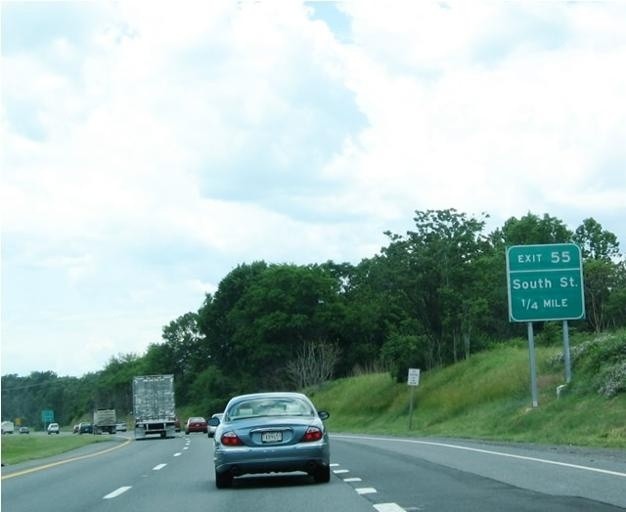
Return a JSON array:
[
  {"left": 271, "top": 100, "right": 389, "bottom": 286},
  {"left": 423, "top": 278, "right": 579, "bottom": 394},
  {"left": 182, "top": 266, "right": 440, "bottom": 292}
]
[
  {"left": 18, "top": 426, "right": 30, "bottom": 434},
  {"left": 205, "top": 389, "right": 337, "bottom": 489},
  {"left": 171, "top": 412, "right": 226, "bottom": 438},
  {"left": 73, "top": 420, "right": 128, "bottom": 434}
]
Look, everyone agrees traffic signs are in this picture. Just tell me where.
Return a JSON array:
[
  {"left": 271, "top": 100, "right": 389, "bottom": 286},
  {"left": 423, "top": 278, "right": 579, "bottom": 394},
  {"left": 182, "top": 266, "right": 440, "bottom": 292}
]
[
  {"left": 40, "top": 410, "right": 55, "bottom": 424},
  {"left": 504, "top": 241, "right": 589, "bottom": 324}
]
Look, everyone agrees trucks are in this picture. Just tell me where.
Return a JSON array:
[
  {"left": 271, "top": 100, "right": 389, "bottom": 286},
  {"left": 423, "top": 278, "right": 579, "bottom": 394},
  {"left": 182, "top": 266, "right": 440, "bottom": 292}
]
[
  {"left": 91, "top": 407, "right": 116, "bottom": 435},
  {"left": 1, "top": 420, "right": 14, "bottom": 434},
  {"left": 127, "top": 373, "right": 178, "bottom": 440}
]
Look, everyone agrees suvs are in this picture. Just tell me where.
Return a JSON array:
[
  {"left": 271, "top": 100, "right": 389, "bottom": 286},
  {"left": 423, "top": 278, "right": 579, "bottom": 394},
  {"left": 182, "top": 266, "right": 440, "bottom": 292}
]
[{"left": 46, "top": 423, "right": 59, "bottom": 434}]
